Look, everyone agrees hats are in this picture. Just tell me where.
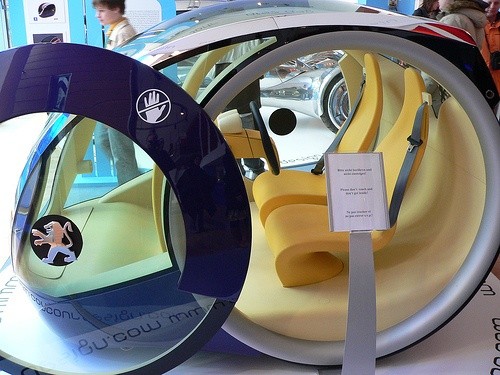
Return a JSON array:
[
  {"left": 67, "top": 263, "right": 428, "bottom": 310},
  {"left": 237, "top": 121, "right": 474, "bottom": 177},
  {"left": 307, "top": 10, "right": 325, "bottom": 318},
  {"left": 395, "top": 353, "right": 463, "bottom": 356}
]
[{"left": 465, "top": 0, "right": 490, "bottom": 10}]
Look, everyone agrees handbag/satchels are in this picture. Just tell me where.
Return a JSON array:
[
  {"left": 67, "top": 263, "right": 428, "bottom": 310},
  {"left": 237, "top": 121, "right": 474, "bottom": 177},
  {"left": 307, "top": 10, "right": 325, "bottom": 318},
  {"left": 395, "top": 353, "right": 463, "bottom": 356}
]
[{"left": 490, "top": 51, "right": 500, "bottom": 70}]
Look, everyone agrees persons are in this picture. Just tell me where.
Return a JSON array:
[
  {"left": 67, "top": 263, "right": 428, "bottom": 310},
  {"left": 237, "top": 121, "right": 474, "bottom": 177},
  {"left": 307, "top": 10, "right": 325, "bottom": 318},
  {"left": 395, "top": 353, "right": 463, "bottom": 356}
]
[
  {"left": 412, "top": 1, "right": 500, "bottom": 94},
  {"left": 213, "top": 39, "right": 269, "bottom": 179},
  {"left": 91, "top": 0, "right": 139, "bottom": 185}
]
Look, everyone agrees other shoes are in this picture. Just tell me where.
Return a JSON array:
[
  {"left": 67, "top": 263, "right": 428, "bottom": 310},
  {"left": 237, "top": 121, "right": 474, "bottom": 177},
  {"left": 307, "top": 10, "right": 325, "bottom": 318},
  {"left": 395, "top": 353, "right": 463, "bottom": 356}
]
[{"left": 245, "top": 170, "right": 269, "bottom": 181}]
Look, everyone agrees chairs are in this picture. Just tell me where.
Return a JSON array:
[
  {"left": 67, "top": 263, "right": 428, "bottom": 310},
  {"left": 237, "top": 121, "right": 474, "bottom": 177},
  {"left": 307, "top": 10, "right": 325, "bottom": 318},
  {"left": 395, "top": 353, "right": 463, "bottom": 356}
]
[{"left": 253, "top": 55, "right": 421, "bottom": 290}]
